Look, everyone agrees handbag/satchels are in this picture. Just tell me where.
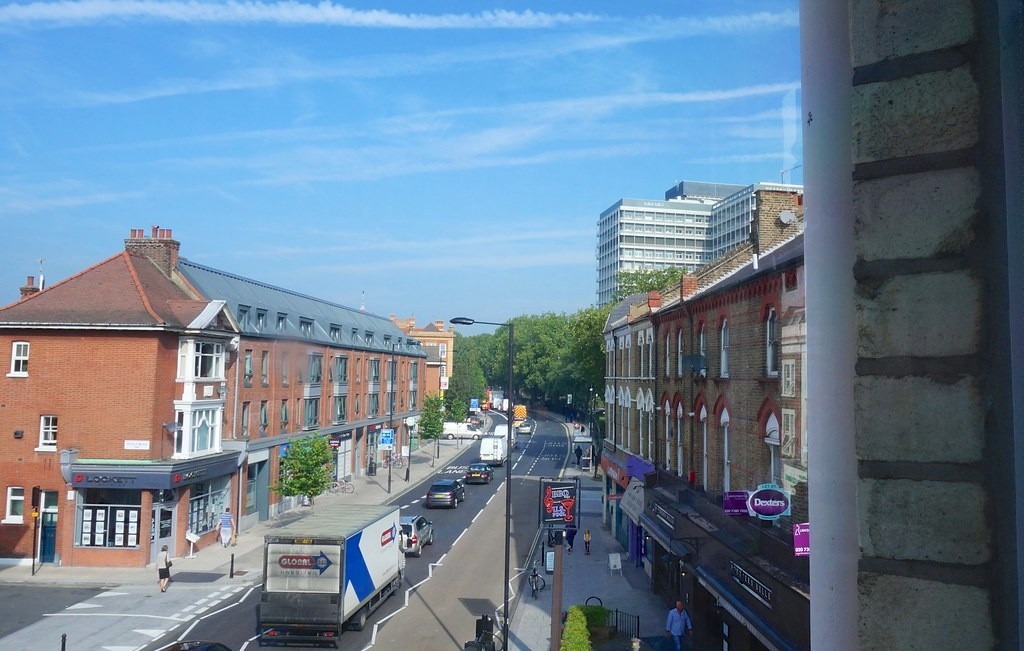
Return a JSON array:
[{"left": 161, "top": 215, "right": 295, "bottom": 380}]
[{"left": 166, "top": 561, "right": 173, "bottom": 568}]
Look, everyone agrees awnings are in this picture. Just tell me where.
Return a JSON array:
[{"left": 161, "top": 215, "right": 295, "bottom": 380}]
[{"left": 619, "top": 477, "right": 644, "bottom": 526}]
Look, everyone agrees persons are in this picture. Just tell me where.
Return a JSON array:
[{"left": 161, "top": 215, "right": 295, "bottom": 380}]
[
  {"left": 216, "top": 508, "right": 236, "bottom": 548},
  {"left": 575, "top": 445, "right": 582, "bottom": 465},
  {"left": 565, "top": 525, "right": 577, "bottom": 554},
  {"left": 158, "top": 545, "right": 170, "bottom": 592},
  {"left": 584, "top": 529, "right": 591, "bottom": 555},
  {"left": 666, "top": 601, "right": 693, "bottom": 651},
  {"left": 589, "top": 445, "right": 595, "bottom": 463}
]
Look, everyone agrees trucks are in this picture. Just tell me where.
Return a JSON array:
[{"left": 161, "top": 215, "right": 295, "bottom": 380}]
[
  {"left": 443, "top": 421, "right": 483, "bottom": 440},
  {"left": 258, "top": 503, "right": 412, "bottom": 649},
  {"left": 479, "top": 389, "right": 527, "bottom": 428},
  {"left": 479, "top": 434, "right": 508, "bottom": 467},
  {"left": 494, "top": 423, "right": 519, "bottom": 449}
]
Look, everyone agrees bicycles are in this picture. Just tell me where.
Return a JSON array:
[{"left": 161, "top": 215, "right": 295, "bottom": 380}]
[
  {"left": 528, "top": 560, "right": 546, "bottom": 599},
  {"left": 330, "top": 479, "right": 355, "bottom": 494},
  {"left": 383, "top": 453, "right": 408, "bottom": 469}
]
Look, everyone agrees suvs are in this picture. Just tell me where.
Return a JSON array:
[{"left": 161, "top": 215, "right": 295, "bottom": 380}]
[{"left": 400, "top": 515, "right": 434, "bottom": 557}]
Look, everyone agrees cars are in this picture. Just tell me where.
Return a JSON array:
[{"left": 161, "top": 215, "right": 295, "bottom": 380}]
[
  {"left": 152, "top": 639, "right": 232, "bottom": 651},
  {"left": 465, "top": 463, "right": 494, "bottom": 485},
  {"left": 426, "top": 479, "right": 465, "bottom": 508},
  {"left": 518, "top": 422, "right": 532, "bottom": 435}
]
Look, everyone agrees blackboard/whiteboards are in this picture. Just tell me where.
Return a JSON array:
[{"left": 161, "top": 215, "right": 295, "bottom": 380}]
[{"left": 609, "top": 553, "right": 622, "bottom": 570}]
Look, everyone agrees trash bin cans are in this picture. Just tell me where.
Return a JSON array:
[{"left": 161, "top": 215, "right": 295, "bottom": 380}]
[{"left": 369, "top": 462, "right": 376, "bottom": 476}]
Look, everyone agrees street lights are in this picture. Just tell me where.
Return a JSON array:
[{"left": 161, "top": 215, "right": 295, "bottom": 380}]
[
  {"left": 436, "top": 348, "right": 458, "bottom": 459},
  {"left": 450, "top": 316, "right": 515, "bottom": 651},
  {"left": 386, "top": 342, "right": 420, "bottom": 494}
]
